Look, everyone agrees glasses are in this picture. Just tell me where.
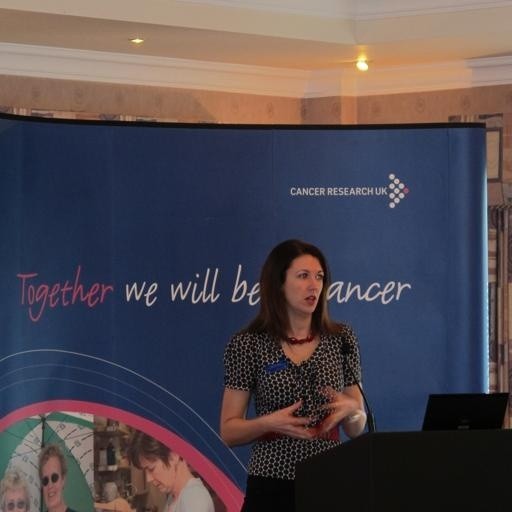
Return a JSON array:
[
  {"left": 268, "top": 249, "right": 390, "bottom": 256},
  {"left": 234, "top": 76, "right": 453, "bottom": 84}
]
[
  {"left": 41, "top": 473, "right": 60, "bottom": 486},
  {"left": 5, "top": 501, "right": 26, "bottom": 510}
]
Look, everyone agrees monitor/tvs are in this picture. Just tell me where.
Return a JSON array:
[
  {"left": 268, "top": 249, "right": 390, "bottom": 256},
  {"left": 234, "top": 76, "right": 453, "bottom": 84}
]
[{"left": 421, "top": 392, "right": 510, "bottom": 431}]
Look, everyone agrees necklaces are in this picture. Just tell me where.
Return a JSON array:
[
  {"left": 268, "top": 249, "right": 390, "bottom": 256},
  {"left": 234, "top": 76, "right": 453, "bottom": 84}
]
[{"left": 282, "top": 326, "right": 317, "bottom": 345}]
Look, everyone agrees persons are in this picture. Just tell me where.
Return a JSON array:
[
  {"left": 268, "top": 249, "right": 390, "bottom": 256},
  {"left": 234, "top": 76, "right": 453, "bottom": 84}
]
[
  {"left": 217, "top": 238, "right": 368, "bottom": 511},
  {"left": 38, "top": 445, "right": 76, "bottom": 511},
  {"left": 126, "top": 430, "right": 215, "bottom": 511},
  {"left": 1, "top": 471, "right": 32, "bottom": 511}
]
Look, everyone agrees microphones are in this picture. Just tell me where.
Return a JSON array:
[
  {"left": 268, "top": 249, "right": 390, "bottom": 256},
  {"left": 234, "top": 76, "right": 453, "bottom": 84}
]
[{"left": 340, "top": 341, "right": 375, "bottom": 433}]
